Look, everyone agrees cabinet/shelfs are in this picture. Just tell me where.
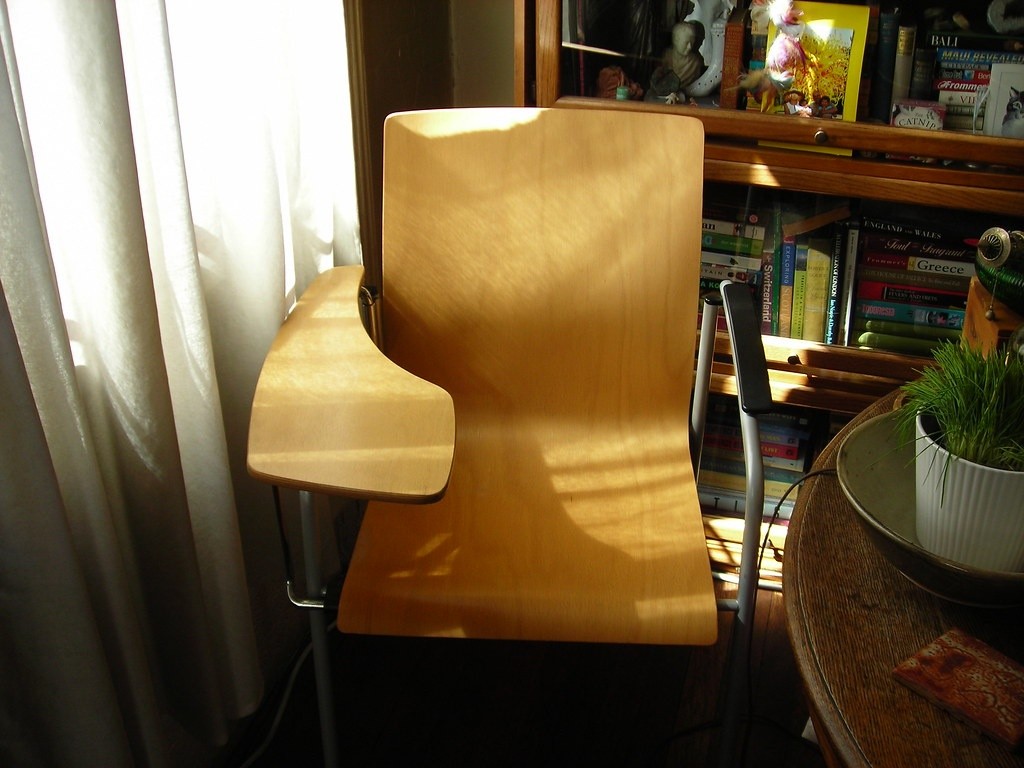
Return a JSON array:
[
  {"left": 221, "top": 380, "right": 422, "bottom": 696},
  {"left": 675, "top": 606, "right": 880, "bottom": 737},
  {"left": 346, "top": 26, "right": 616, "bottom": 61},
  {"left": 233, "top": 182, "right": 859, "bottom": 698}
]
[{"left": 512, "top": 0, "right": 1024, "bottom": 595}]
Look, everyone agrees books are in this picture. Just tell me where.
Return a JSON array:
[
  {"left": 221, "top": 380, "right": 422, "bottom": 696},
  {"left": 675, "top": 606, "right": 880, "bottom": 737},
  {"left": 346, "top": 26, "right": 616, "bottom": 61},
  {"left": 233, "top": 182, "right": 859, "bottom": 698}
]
[
  {"left": 697, "top": 185, "right": 1024, "bottom": 362},
  {"left": 892, "top": 626, "right": 1024, "bottom": 750},
  {"left": 698, "top": 397, "right": 853, "bottom": 519},
  {"left": 720, "top": 0, "right": 1024, "bottom": 168}
]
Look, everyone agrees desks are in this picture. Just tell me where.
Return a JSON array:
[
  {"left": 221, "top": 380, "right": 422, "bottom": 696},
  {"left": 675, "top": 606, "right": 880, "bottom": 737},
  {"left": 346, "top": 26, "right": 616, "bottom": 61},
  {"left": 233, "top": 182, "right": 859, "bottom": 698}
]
[{"left": 784, "top": 375, "right": 1024, "bottom": 768}]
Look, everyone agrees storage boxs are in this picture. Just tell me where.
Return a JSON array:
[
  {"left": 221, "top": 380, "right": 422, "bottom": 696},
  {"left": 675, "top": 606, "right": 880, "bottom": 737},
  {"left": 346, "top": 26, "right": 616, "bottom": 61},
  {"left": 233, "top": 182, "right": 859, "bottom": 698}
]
[{"left": 885, "top": 98, "right": 947, "bottom": 164}]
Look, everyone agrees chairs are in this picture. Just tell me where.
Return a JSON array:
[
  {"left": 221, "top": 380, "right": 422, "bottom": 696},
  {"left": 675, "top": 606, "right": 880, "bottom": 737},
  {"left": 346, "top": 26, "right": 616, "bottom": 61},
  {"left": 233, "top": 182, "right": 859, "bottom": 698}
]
[{"left": 243, "top": 105, "right": 776, "bottom": 768}]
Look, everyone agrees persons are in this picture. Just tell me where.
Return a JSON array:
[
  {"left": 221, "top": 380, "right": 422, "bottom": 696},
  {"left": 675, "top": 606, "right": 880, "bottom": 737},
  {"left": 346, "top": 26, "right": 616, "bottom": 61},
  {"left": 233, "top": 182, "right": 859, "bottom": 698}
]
[{"left": 644, "top": 20, "right": 708, "bottom": 103}]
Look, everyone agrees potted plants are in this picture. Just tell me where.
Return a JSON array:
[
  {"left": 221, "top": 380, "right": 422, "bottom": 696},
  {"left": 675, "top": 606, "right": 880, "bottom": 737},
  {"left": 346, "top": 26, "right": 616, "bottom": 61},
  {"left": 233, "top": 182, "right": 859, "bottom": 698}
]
[{"left": 860, "top": 334, "right": 1024, "bottom": 572}]
